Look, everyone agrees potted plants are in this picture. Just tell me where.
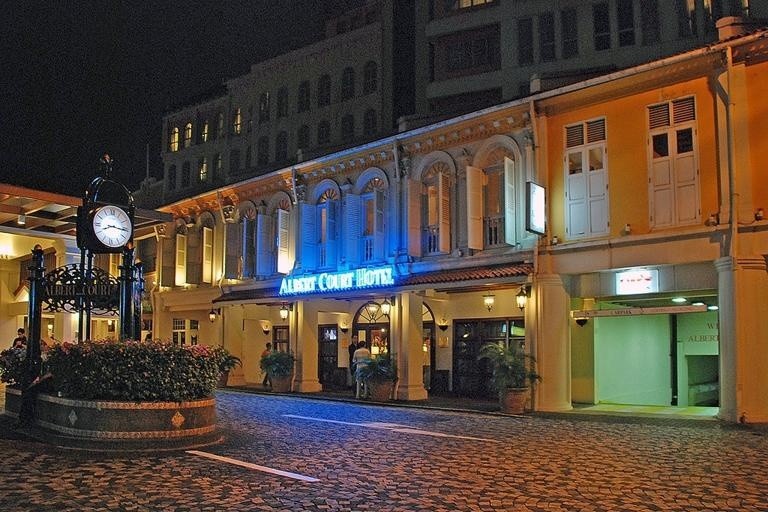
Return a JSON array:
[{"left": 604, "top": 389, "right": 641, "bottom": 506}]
[
  {"left": 478, "top": 338, "right": 539, "bottom": 414},
  {"left": 260, "top": 351, "right": 297, "bottom": 393},
  {"left": 353, "top": 352, "right": 400, "bottom": 402}
]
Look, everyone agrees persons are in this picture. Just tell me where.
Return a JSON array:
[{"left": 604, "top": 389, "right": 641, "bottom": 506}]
[
  {"left": 351, "top": 341, "right": 372, "bottom": 399},
  {"left": 422, "top": 337, "right": 433, "bottom": 392},
  {"left": 49, "top": 337, "right": 57, "bottom": 346},
  {"left": 12, "top": 328, "right": 27, "bottom": 351},
  {"left": 261, "top": 342, "right": 274, "bottom": 387},
  {"left": 348, "top": 332, "right": 361, "bottom": 394}
]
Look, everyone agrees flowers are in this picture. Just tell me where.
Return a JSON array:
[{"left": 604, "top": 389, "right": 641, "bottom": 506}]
[{"left": 6, "top": 340, "right": 233, "bottom": 401}]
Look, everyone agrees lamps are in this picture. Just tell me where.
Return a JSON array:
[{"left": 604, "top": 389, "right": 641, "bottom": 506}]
[
  {"left": 263, "top": 329, "right": 270, "bottom": 335},
  {"left": 340, "top": 327, "right": 350, "bottom": 333},
  {"left": 208, "top": 308, "right": 216, "bottom": 324},
  {"left": 438, "top": 323, "right": 449, "bottom": 331},
  {"left": 482, "top": 287, "right": 496, "bottom": 312},
  {"left": 380, "top": 296, "right": 391, "bottom": 315},
  {"left": 280, "top": 302, "right": 289, "bottom": 321},
  {"left": 516, "top": 284, "right": 527, "bottom": 311}
]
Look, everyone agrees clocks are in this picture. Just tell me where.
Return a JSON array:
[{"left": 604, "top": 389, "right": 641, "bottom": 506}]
[{"left": 91, "top": 203, "right": 136, "bottom": 247}]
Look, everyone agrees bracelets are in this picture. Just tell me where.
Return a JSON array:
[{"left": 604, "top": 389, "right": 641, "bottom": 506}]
[{"left": 20, "top": 343, "right": 22, "bottom": 347}]
